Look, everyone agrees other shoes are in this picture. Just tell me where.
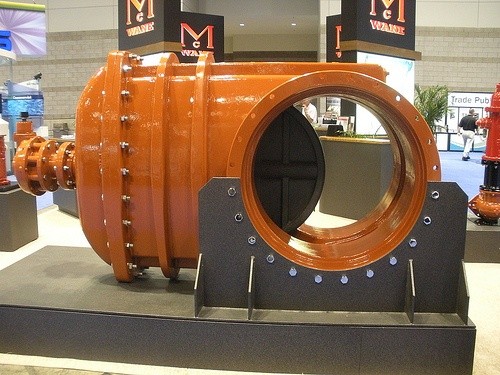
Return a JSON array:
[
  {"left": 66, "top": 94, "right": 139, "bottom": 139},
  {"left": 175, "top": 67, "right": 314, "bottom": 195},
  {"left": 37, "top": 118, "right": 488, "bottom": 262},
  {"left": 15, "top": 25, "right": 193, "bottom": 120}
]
[
  {"left": 462, "top": 157, "right": 468, "bottom": 161},
  {"left": 466, "top": 156, "right": 470, "bottom": 159}
]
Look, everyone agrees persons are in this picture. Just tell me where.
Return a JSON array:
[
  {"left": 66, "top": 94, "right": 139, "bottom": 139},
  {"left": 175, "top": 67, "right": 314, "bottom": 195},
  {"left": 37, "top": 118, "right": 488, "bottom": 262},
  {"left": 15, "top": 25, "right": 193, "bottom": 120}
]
[
  {"left": 458, "top": 109, "right": 479, "bottom": 161},
  {"left": 302, "top": 99, "right": 317, "bottom": 125}
]
